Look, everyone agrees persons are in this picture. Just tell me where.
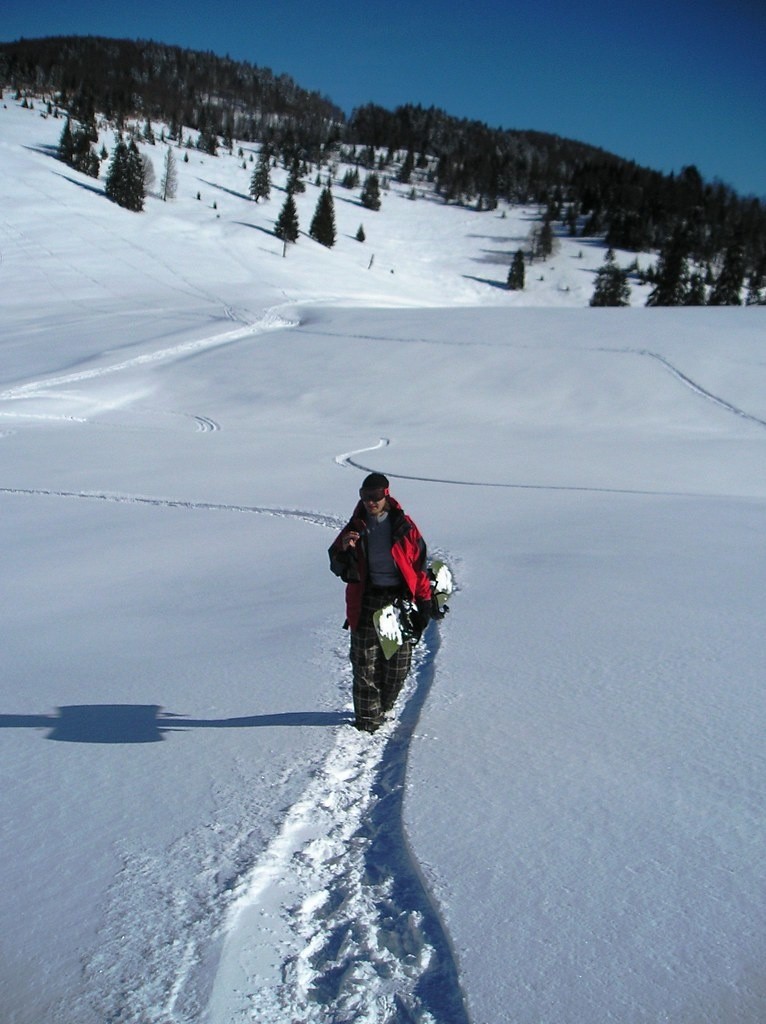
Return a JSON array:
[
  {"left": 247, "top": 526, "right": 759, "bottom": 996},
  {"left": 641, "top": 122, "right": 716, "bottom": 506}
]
[{"left": 326, "top": 474, "right": 431, "bottom": 732}]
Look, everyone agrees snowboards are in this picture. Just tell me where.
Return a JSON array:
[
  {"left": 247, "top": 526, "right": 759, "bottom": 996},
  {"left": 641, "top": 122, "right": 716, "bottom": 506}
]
[{"left": 372, "top": 559, "right": 456, "bottom": 662}]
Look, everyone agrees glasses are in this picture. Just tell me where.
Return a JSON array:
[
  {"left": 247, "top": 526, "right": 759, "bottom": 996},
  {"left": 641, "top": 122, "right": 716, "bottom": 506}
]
[{"left": 359, "top": 488, "right": 389, "bottom": 502}]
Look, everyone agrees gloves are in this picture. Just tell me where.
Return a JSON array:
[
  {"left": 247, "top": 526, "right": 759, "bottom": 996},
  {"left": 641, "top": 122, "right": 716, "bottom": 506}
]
[{"left": 420, "top": 599, "right": 432, "bottom": 620}]
